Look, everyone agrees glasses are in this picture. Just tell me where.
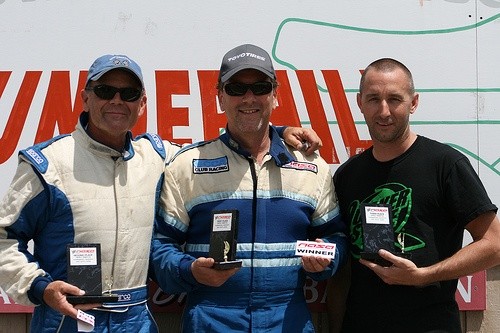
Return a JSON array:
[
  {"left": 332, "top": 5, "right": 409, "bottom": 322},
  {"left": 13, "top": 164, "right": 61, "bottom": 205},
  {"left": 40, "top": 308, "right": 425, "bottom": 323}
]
[
  {"left": 85, "top": 83, "right": 142, "bottom": 103},
  {"left": 224, "top": 81, "right": 274, "bottom": 96}
]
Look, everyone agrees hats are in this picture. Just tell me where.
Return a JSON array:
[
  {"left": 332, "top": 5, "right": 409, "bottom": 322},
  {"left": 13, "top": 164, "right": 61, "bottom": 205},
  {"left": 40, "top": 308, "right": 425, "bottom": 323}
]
[
  {"left": 86, "top": 54, "right": 144, "bottom": 84},
  {"left": 219, "top": 43, "right": 274, "bottom": 80}
]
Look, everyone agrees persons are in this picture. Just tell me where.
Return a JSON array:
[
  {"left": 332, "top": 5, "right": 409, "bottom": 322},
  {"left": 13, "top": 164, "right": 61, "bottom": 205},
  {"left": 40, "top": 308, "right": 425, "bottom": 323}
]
[
  {"left": 148, "top": 45, "right": 348, "bottom": 333},
  {"left": 332, "top": 59, "right": 500, "bottom": 333},
  {"left": 0, "top": 55, "right": 323, "bottom": 333}
]
